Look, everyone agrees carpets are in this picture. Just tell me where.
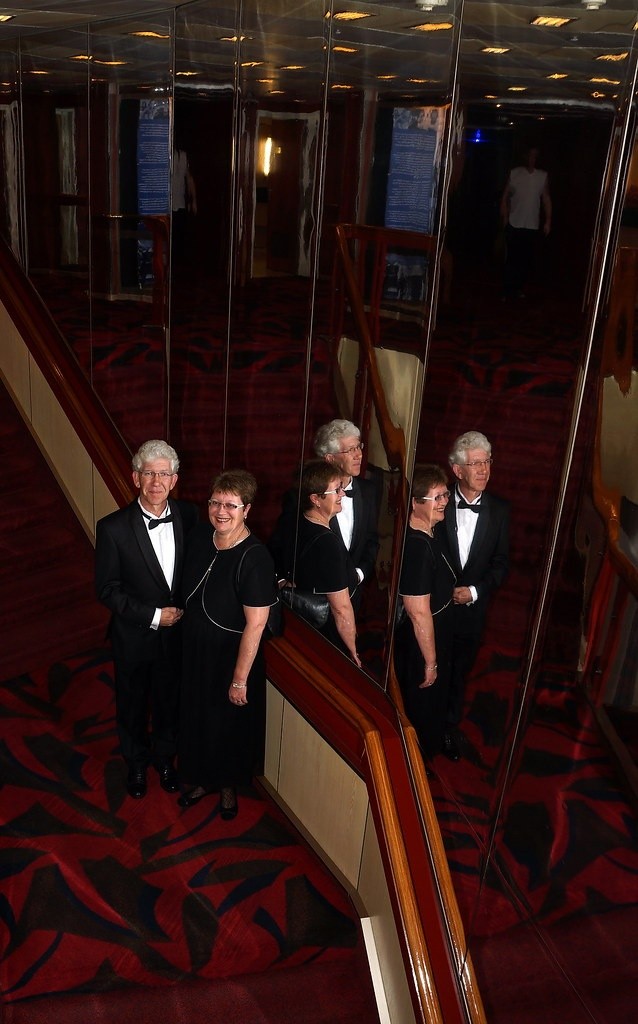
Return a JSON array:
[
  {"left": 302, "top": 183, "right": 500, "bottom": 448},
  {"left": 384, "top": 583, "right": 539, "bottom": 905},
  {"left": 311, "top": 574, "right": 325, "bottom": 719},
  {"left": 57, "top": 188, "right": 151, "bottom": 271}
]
[
  {"left": 469, "top": 655, "right": 638, "bottom": 944},
  {"left": 23, "top": 267, "right": 93, "bottom": 365},
  {"left": 427, "top": 319, "right": 584, "bottom": 400},
  {"left": 167, "top": 310, "right": 230, "bottom": 376},
  {"left": 87, "top": 298, "right": 168, "bottom": 368},
  {"left": 419, "top": 648, "right": 529, "bottom": 935},
  {"left": 312, "top": 611, "right": 390, "bottom": 692},
  {"left": 0, "top": 647, "right": 364, "bottom": 1006},
  {"left": 309, "top": 303, "right": 427, "bottom": 384},
  {"left": 231, "top": 303, "right": 310, "bottom": 375}
]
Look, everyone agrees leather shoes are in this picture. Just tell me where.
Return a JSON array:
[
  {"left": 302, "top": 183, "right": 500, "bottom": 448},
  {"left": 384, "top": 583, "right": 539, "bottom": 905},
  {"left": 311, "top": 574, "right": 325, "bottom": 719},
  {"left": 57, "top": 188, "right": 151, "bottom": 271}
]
[
  {"left": 153, "top": 759, "right": 179, "bottom": 795},
  {"left": 125, "top": 763, "right": 149, "bottom": 800},
  {"left": 440, "top": 730, "right": 461, "bottom": 762}
]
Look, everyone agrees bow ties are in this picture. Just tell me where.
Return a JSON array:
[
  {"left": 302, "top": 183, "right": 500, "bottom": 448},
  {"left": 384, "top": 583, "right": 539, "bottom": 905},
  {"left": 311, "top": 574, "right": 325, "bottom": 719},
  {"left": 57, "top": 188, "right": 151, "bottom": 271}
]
[
  {"left": 458, "top": 499, "right": 482, "bottom": 513},
  {"left": 343, "top": 490, "right": 354, "bottom": 498},
  {"left": 148, "top": 513, "right": 175, "bottom": 531}
]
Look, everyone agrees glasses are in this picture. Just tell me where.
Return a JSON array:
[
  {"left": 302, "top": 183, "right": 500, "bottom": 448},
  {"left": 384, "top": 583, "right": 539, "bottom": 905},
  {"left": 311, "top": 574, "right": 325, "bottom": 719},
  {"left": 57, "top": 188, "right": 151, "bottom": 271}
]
[
  {"left": 336, "top": 443, "right": 364, "bottom": 455},
  {"left": 460, "top": 459, "right": 493, "bottom": 467},
  {"left": 316, "top": 481, "right": 343, "bottom": 495},
  {"left": 138, "top": 471, "right": 174, "bottom": 478},
  {"left": 208, "top": 499, "right": 244, "bottom": 511},
  {"left": 419, "top": 490, "right": 450, "bottom": 503}
]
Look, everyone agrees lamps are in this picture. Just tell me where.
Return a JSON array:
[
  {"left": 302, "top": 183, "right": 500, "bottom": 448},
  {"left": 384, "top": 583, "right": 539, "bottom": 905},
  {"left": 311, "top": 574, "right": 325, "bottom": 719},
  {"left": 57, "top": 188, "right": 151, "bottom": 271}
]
[{"left": 264, "top": 134, "right": 272, "bottom": 179}]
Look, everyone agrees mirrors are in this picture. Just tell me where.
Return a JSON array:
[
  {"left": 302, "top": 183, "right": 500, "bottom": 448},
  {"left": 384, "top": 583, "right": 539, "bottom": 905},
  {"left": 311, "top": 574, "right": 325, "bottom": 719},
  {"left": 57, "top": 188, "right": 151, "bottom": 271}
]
[{"left": 0, "top": 0, "right": 638, "bottom": 1024}]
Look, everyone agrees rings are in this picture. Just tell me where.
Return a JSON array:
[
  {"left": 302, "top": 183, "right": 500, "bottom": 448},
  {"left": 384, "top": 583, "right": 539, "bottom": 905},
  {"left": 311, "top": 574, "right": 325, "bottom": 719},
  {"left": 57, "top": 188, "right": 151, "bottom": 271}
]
[
  {"left": 238, "top": 701, "right": 242, "bottom": 705},
  {"left": 429, "top": 682, "right": 432, "bottom": 686},
  {"left": 174, "top": 618, "right": 177, "bottom": 622}
]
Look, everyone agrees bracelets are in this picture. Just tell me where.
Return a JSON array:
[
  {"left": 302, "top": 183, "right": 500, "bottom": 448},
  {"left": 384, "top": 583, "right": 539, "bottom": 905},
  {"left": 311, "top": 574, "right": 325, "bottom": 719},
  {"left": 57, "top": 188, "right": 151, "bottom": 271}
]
[{"left": 424, "top": 662, "right": 438, "bottom": 671}]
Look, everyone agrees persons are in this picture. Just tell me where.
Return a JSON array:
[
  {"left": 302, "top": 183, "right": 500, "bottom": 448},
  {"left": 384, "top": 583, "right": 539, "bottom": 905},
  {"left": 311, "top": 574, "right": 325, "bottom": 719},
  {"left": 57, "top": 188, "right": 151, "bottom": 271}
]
[
  {"left": 94, "top": 440, "right": 202, "bottom": 798},
  {"left": 498, "top": 146, "right": 552, "bottom": 301},
  {"left": 274, "top": 461, "right": 363, "bottom": 668},
  {"left": 315, "top": 418, "right": 387, "bottom": 648},
  {"left": 434, "top": 430, "right": 510, "bottom": 761},
  {"left": 169, "top": 142, "right": 198, "bottom": 276},
  {"left": 177, "top": 470, "right": 276, "bottom": 823},
  {"left": 390, "top": 464, "right": 458, "bottom": 778}
]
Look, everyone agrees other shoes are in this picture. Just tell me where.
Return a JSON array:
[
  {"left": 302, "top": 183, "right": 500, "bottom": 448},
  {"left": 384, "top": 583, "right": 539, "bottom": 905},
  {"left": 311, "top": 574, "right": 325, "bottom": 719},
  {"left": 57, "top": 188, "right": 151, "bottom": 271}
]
[
  {"left": 220, "top": 786, "right": 238, "bottom": 820},
  {"left": 176, "top": 786, "right": 218, "bottom": 808},
  {"left": 426, "top": 768, "right": 432, "bottom": 773}
]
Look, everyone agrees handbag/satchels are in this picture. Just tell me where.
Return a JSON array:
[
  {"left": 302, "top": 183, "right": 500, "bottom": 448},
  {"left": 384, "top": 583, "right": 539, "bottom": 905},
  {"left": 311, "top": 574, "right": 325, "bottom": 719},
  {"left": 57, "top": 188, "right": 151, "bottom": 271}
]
[
  {"left": 281, "top": 585, "right": 330, "bottom": 630},
  {"left": 235, "top": 544, "right": 285, "bottom": 642}
]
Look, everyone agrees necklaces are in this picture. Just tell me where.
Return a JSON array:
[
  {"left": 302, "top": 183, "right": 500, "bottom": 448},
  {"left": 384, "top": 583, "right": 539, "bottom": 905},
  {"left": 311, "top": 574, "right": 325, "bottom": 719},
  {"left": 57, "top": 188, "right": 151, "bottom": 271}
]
[
  {"left": 304, "top": 511, "right": 329, "bottom": 526},
  {"left": 409, "top": 517, "right": 434, "bottom": 537}
]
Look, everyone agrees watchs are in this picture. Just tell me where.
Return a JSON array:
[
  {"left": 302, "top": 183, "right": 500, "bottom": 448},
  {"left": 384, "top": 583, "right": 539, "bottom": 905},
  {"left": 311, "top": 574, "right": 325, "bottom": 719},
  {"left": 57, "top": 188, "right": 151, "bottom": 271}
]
[{"left": 231, "top": 680, "right": 247, "bottom": 688}]
